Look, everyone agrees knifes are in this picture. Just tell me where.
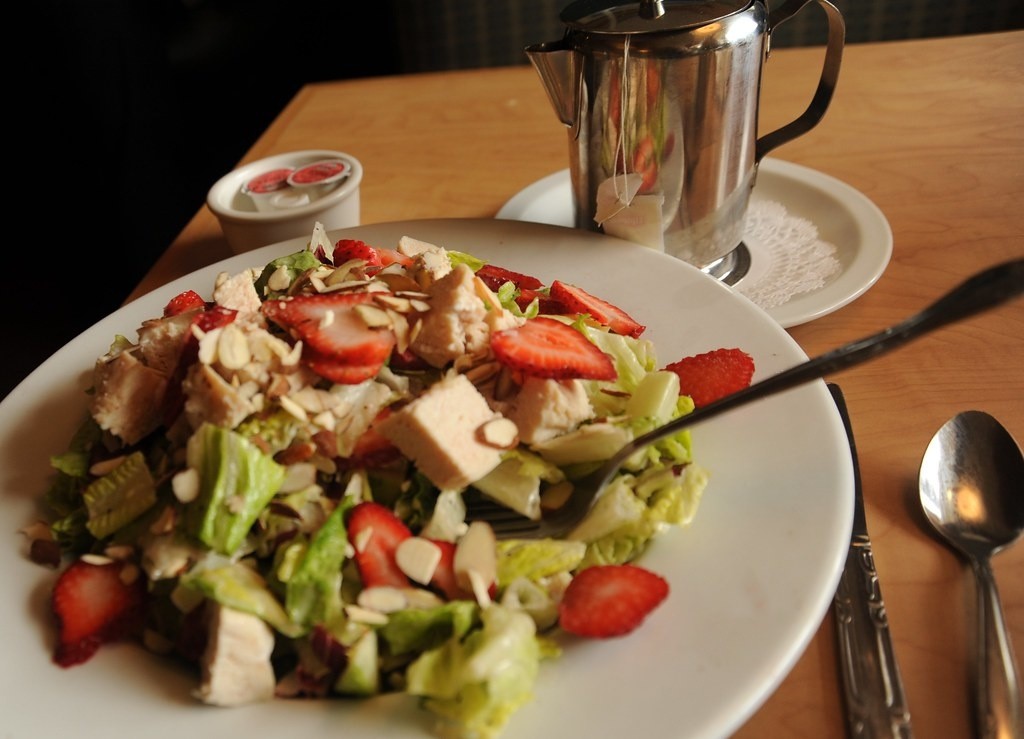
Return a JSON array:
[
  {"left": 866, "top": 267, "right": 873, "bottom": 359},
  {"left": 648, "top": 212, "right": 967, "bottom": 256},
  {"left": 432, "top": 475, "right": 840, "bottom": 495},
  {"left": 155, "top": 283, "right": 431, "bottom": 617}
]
[{"left": 820, "top": 382, "right": 915, "bottom": 739}]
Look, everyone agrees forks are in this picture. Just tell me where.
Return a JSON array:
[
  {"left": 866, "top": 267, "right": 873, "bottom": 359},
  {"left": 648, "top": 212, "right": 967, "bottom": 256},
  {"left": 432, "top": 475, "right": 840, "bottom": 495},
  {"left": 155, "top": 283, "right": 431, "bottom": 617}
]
[{"left": 453, "top": 257, "right": 1024, "bottom": 544}]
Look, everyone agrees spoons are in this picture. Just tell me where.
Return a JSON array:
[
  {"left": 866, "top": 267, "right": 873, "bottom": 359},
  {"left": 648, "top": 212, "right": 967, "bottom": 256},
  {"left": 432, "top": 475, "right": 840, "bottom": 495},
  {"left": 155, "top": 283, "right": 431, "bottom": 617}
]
[{"left": 916, "top": 408, "right": 1024, "bottom": 739}]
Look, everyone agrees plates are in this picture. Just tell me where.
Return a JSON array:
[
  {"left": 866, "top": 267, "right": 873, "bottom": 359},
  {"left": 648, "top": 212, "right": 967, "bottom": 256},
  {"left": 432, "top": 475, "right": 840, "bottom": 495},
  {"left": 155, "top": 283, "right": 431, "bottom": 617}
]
[
  {"left": 497, "top": 152, "right": 895, "bottom": 333},
  {"left": 2, "top": 221, "right": 858, "bottom": 739}
]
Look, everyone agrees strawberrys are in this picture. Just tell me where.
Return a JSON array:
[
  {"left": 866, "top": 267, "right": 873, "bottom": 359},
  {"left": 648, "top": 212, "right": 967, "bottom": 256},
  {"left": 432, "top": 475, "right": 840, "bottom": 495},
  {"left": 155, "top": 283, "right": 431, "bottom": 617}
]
[{"left": 54, "top": 240, "right": 756, "bottom": 667}]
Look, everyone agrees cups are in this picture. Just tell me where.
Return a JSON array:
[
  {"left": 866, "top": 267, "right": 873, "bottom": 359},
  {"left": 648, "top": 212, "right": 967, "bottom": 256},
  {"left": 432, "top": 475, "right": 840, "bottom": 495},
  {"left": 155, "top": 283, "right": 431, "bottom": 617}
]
[{"left": 208, "top": 149, "right": 365, "bottom": 260}]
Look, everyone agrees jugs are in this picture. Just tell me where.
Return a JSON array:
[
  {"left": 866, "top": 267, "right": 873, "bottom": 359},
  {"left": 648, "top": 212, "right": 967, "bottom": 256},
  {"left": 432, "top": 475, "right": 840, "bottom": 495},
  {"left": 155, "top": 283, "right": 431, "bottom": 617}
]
[{"left": 526, "top": 0, "right": 846, "bottom": 288}]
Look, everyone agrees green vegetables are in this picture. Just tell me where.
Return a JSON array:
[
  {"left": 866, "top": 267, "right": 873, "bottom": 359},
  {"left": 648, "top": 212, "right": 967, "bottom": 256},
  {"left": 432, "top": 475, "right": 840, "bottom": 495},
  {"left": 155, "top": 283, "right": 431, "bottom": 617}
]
[{"left": 50, "top": 248, "right": 709, "bottom": 739}]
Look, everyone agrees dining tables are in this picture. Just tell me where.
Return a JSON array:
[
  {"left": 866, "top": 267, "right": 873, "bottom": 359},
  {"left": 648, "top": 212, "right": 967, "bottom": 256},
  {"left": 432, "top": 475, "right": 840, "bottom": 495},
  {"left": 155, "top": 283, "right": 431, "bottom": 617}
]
[{"left": 124, "top": 27, "right": 1024, "bottom": 739}]
